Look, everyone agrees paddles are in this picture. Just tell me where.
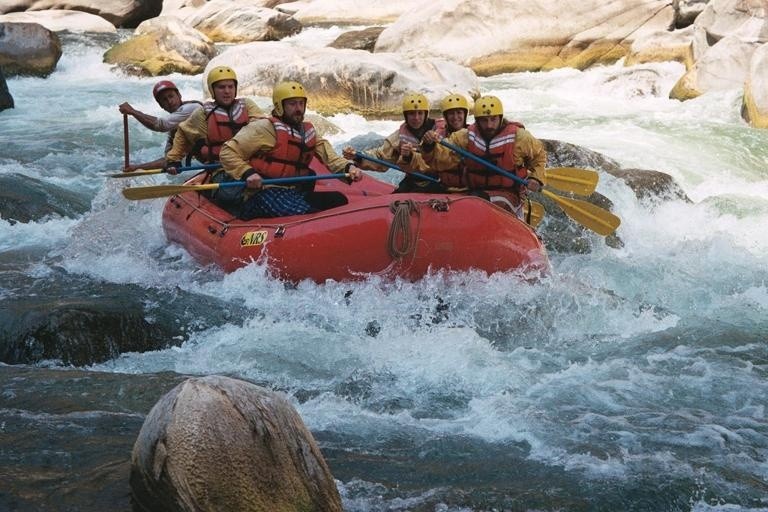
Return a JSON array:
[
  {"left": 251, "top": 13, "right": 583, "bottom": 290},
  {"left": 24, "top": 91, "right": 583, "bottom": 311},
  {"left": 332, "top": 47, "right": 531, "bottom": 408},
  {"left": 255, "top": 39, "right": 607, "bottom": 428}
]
[
  {"left": 104, "top": 164, "right": 222, "bottom": 177},
  {"left": 350, "top": 150, "right": 544, "bottom": 230},
  {"left": 438, "top": 138, "right": 620, "bottom": 236},
  {"left": 123, "top": 173, "right": 351, "bottom": 200},
  {"left": 527, "top": 166, "right": 598, "bottom": 197}
]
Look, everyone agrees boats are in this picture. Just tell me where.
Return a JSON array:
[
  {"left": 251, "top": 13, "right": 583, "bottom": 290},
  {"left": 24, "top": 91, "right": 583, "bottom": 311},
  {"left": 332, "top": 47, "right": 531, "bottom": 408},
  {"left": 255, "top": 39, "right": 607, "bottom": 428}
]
[{"left": 159, "top": 158, "right": 549, "bottom": 295}]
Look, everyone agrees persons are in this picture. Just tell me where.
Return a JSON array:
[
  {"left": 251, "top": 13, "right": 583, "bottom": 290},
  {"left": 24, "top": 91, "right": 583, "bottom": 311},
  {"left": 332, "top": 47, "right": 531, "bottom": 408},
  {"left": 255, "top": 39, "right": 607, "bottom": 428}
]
[
  {"left": 422, "top": 96, "right": 545, "bottom": 220},
  {"left": 119, "top": 80, "right": 204, "bottom": 172},
  {"left": 219, "top": 81, "right": 363, "bottom": 221},
  {"left": 401, "top": 94, "right": 472, "bottom": 194},
  {"left": 164, "top": 67, "right": 264, "bottom": 208},
  {"left": 342, "top": 95, "right": 448, "bottom": 194}
]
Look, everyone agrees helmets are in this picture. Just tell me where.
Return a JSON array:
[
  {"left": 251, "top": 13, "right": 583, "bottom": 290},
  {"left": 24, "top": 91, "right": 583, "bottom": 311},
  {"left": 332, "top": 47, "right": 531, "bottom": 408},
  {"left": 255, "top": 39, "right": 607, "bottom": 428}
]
[
  {"left": 272, "top": 80, "right": 308, "bottom": 117},
  {"left": 207, "top": 66, "right": 237, "bottom": 99},
  {"left": 403, "top": 91, "right": 429, "bottom": 124},
  {"left": 473, "top": 95, "right": 504, "bottom": 131},
  {"left": 440, "top": 94, "right": 468, "bottom": 129},
  {"left": 153, "top": 81, "right": 182, "bottom": 108}
]
